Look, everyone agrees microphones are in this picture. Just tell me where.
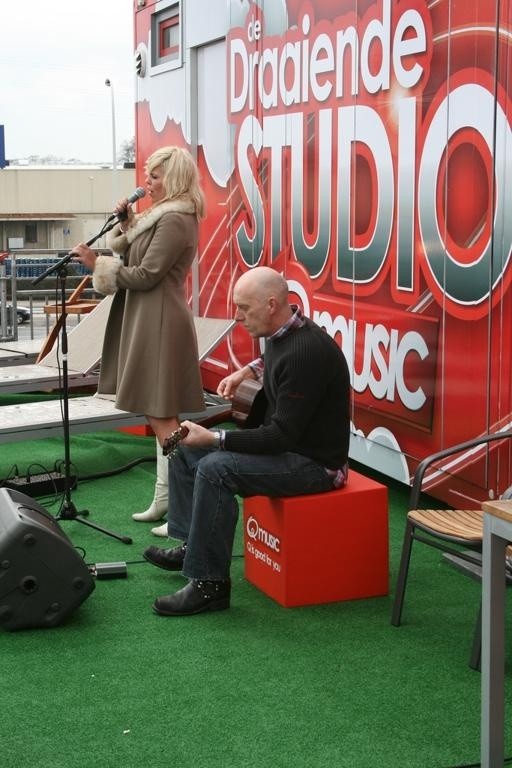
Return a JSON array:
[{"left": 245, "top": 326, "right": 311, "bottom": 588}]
[{"left": 107, "top": 186, "right": 147, "bottom": 222}]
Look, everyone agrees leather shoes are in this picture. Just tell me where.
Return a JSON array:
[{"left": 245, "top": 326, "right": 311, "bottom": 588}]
[{"left": 152, "top": 578, "right": 231, "bottom": 616}]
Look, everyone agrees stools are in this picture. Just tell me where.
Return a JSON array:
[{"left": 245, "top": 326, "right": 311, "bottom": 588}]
[{"left": 244, "top": 466, "right": 391, "bottom": 608}]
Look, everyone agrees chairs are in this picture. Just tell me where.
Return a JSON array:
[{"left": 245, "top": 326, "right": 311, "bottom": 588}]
[{"left": 391, "top": 430, "right": 512, "bottom": 673}]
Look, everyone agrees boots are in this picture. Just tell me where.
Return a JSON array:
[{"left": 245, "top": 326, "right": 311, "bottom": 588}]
[
  {"left": 143, "top": 542, "right": 189, "bottom": 572},
  {"left": 132, "top": 435, "right": 168, "bottom": 537}
]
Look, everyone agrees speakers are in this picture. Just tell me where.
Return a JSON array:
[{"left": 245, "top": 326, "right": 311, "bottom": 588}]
[{"left": 0, "top": 486, "right": 96, "bottom": 632}]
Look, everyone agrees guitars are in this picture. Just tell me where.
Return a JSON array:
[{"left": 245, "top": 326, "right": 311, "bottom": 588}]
[{"left": 163, "top": 374, "right": 267, "bottom": 460}]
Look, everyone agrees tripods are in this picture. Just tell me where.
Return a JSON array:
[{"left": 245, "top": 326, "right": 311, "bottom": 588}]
[{"left": 32, "top": 218, "right": 134, "bottom": 545}]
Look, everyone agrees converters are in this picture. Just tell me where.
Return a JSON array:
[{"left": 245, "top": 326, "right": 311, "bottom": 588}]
[{"left": 96, "top": 560, "right": 129, "bottom": 580}]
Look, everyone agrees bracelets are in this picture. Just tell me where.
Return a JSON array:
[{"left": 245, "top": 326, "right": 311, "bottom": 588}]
[{"left": 213, "top": 431, "right": 221, "bottom": 448}]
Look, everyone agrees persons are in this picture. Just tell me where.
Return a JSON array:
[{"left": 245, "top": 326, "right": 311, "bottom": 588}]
[
  {"left": 142, "top": 266, "right": 350, "bottom": 617},
  {"left": 69, "top": 146, "right": 206, "bottom": 536}
]
